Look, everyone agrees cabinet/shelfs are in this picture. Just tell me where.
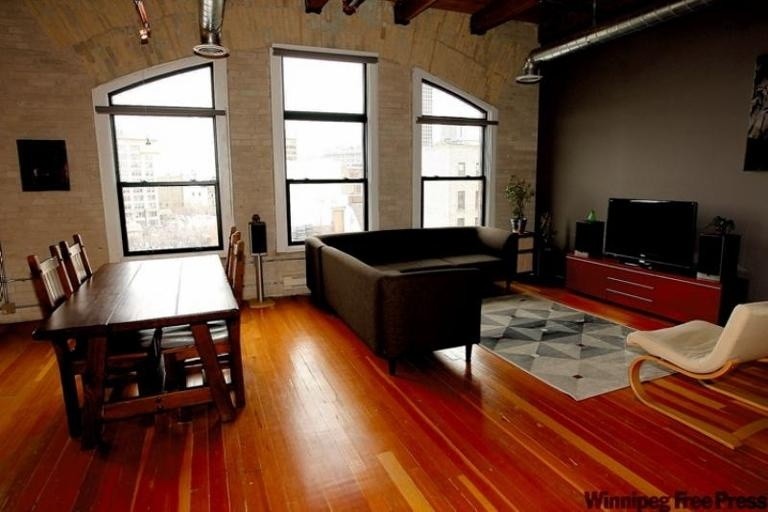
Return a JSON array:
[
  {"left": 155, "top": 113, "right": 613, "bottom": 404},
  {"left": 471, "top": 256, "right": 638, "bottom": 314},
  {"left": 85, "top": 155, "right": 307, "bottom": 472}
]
[{"left": 564, "top": 250, "right": 727, "bottom": 327}]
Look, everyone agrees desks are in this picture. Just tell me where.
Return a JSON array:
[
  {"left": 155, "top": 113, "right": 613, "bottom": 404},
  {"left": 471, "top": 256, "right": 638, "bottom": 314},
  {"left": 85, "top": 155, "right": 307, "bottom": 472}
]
[{"left": 511, "top": 231, "right": 540, "bottom": 280}]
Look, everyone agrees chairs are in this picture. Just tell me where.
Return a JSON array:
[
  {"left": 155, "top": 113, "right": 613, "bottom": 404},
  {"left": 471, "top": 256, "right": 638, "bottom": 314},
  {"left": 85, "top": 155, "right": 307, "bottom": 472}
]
[{"left": 624, "top": 298, "right": 768, "bottom": 451}]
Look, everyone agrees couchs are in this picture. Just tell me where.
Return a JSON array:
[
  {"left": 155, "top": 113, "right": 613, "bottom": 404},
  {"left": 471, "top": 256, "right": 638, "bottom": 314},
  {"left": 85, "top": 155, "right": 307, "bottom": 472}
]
[{"left": 305, "top": 227, "right": 519, "bottom": 376}]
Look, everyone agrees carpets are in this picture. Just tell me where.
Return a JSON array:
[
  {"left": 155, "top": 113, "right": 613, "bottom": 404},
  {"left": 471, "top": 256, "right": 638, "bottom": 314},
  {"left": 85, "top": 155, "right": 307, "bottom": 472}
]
[{"left": 476, "top": 292, "right": 677, "bottom": 402}]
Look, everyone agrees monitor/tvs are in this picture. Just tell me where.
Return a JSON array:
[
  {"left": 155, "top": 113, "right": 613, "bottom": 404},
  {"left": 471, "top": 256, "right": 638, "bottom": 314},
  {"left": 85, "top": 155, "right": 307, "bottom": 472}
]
[{"left": 602, "top": 199, "right": 698, "bottom": 273}]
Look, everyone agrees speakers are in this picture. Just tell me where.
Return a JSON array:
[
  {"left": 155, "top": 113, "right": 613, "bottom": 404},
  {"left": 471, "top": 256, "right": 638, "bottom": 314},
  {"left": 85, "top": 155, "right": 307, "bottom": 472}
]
[
  {"left": 575, "top": 219, "right": 603, "bottom": 258},
  {"left": 696, "top": 233, "right": 721, "bottom": 280},
  {"left": 248, "top": 221, "right": 269, "bottom": 257}
]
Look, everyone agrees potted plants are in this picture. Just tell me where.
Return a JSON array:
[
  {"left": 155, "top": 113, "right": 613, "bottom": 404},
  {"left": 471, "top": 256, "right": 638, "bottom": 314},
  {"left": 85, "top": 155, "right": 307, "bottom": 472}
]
[{"left": 504, "top": 175, "right": 535, "bottom": 234}]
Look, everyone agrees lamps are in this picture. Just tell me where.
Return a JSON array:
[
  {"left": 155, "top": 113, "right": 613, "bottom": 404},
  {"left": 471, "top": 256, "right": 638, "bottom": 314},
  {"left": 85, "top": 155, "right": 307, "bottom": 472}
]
[
  {"left": 195, "top": 1, "right": 228, "bottom": 59},
  {"left": 514, "top": 1, "right": 709, "bottom": 86}
]
